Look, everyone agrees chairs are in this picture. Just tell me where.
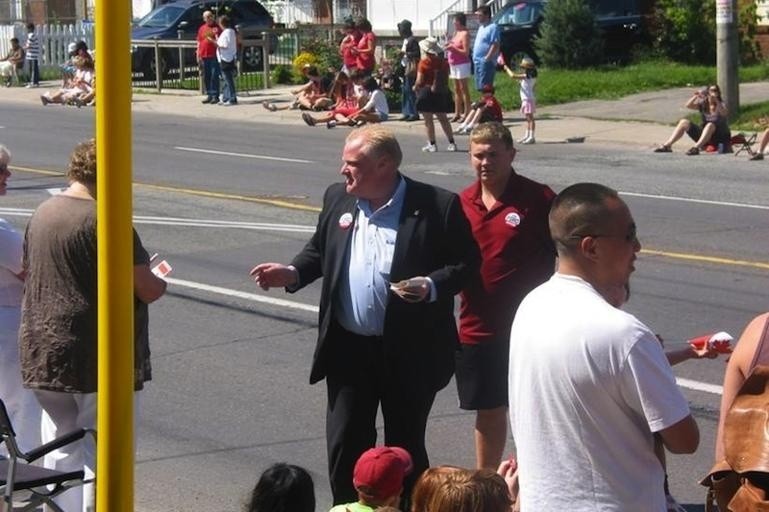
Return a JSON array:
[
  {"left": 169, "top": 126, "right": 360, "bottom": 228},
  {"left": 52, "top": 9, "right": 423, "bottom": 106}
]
[{"left": 0, "top": 400, "right": 97, "bottom": 510}]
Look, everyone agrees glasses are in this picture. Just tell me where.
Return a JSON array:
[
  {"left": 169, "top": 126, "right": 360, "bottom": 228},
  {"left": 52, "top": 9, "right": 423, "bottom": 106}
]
[{"left": 579, "top": 222, "right": 637, "bottom": 244}]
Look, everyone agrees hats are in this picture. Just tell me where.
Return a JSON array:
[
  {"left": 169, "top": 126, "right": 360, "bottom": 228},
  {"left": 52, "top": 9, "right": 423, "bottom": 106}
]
[
  {"left": 300, "top": 63, "right": 311, "bottom": 72},
  {"left": 519, "top": 58, "right": 534, "bottom": 69},
  {"left": 418, "top": 37, "right": 443, "bottom": 54},
  {"left": 353, "top": 446, "right": 413, "bottom": 496},
  {"left": 398, "top": 20, "right": 412, "bottom": 34},
  {"left": 477, "top": 83, "right": 494, "bottom": 93}
]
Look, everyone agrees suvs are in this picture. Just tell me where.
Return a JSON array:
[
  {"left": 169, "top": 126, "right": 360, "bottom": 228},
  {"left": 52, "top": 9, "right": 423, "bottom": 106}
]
[{"left": 130, "top": 0, "right": 279, "bottom": 79}]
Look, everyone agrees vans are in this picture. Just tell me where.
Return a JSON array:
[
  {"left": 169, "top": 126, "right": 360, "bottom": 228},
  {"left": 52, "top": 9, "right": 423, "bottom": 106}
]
[{"left": 491, "top": 0, "right": 649, "bottom": 72}]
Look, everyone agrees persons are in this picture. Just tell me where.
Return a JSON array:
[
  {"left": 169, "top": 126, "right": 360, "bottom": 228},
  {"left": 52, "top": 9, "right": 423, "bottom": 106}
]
[
  {"left": 197, "top": 12, "right": 221, "bottom": 104},
  {"left": 714, "top": 312, "right": 769, "bottom": 463},
  {"left": 0, "top": 142, "right": 42, "bottom": 488},
  {"left": 507, "top": 184, "right": 700, "bottom": 512},
  {"left": 653, "top": 84, "right": 733, "bottom": 155},
  {"left": 0, "top": 23, "right": 97, "bottom": 108},
  {"left": 608, "top": 284, "right": 718, "bottom": 512},
  {"left": 19, "top": 140, "right": 168, "bottom": 512},
  {"left": 251, "top": 127, "right": 482, "bottom": 512},
  {"left": 261, "top": 5, "right": 537, "bottom": 151},
  {"left": 207, "top": 16, "right": 239, "bottom": 105},
  {"left": 748, "top": 128, "right": 769, "bottom": 161},
  {"left": 455, "top": 123, "right": 559, "bottom": 472},
  {"left": 243, "top": 447, "right": 520, "bottom": 512}
]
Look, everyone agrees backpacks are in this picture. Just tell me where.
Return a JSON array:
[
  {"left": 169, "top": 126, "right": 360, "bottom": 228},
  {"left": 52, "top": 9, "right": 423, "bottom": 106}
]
[{"left": 428, "top": 59, "right": 448, "bottom": 97}]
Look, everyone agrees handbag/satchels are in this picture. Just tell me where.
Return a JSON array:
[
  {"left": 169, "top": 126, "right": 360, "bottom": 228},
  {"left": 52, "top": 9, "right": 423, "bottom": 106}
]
[
  {"left": 697, "top": 364, "right": 769, "bottom": 512},
  {"left": 198, "top": 58, "right": 204, "bottom": 76},
  {"left": 719, "top": 140, "right": 732, "bottom": 153},
  {"left": 394, "top": 57, "right": 417, "bottom": 78},
  {"left": 221, "top": 59, "right": 234, "bottom": 71}
]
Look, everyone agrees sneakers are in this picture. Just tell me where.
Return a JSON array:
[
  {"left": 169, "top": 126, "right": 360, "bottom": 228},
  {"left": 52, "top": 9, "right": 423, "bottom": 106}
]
[
  {"left": 202, "top": 95, "right": 237, "bottom": 106},
  {"left": 262, "top": 100, "right": 277, "bottom": 111},
  {"left": 290, "top": 101, "right": 367, "bottom": 128},
  {"left": 516, "top": 136, "right": 534, "bottom": 144},
  {"left": 3, "top": 77, "right": 38, "bottom": 88},
  {"left": 449, "top": 114, "right": 472, "bottom": 135},
  {"left": 398, "top": 113, "right": 418, "bottom": 120},
  {"left": 688, "top": 147, "right": 699, "bottom": 155},
  {"left": 41, "top": 94, "right": 94, "bottom": 108},
  {"left": 750, "top": 151, "right": 764, "bottom": 160},
  {"left": 654, "top": 144, "right": 673, "bottom": 152},
  {"left": 422, "top": 141, "right": 457, "bottom": 152}
]
